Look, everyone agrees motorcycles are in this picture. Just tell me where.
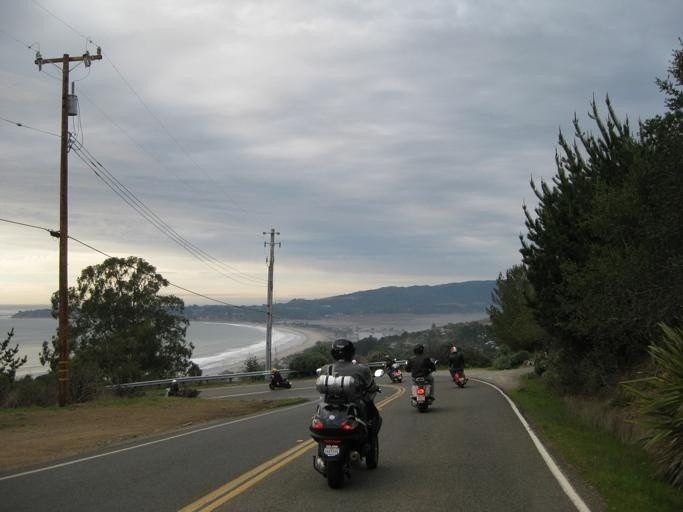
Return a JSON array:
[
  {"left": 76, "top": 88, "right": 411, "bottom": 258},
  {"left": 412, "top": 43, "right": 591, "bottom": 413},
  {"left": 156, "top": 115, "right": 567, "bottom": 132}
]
[
  {"left": 405, "top": 359, "right": 438, "bottom": 411},
  {"left": 449, "top": 368, "right": 468, "bottom": 388},
  {"left": 382, "top": 359, "right": 403, "bottom": 383},
  {"left": 309, "top": 388, "right": 383, "bottom": 488},
  {"left": 269, "top": 377, "right": 292, "bottom": 390}
]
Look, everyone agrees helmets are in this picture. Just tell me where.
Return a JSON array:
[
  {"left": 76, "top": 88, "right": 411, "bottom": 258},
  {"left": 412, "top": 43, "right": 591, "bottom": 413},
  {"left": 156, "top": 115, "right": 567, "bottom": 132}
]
[
  {"left": 450, "top": 346, "right": 457, "bottom": 353},
  {"left": 330, "top": 339, "right": 355, "bottom": 362},
  {"left": 413, "top": 344, "right": 425, "bottom": 355}
]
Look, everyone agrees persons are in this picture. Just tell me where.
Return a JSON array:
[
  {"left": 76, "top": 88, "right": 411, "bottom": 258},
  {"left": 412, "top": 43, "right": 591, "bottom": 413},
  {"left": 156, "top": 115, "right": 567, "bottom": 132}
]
[
  {"left": 448, "top": 346, "right": 465, "bottom": 382},
  {"left": 272, "top": 369, "right": 282, "bottom": 386},
  {"left": 405, "top": 344, "right": 436, "bottom": 399},
  {"left": 321, "top": 339, "right": 379, "bottom": 450},
  {"left": 170, "top": 379, "right": 179, "bottom": 395},
  {"left": 384, "top": 355, "right": 395, "bottom": 377}
]
